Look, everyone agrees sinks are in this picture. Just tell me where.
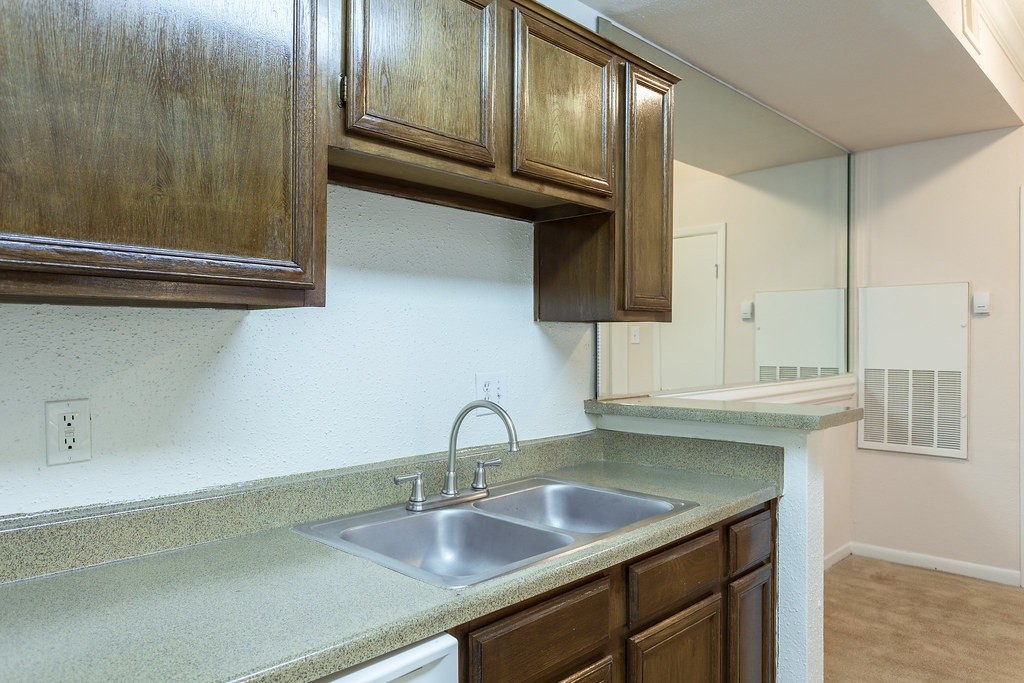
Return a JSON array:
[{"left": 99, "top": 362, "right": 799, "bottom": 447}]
[
  {"left": 465, "top": 473, "right": 701, "bottom": 536},
  {"left": 286, "top": 494, "right": 578, "bottom": 591}
]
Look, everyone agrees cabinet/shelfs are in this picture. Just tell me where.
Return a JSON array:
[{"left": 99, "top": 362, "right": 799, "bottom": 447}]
[
  {"left": 532, "top": 47, "right": 683, "bottom": 323},
  {"left": 327, "top": 0, "right": 617, "bottom": 224},
  {"left": 0, "top": 0, "right": 328, "bottom": 310},
  {"left": 445, "top": 497, "right": 777, "bottom": 683}
]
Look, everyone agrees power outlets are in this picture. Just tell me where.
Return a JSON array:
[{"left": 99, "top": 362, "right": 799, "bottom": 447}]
[
  {"left": 475, "top": 370, "right": 510, "bottom": 416},
  {"left": 45, "top": 397, "right": 93, "bottom": 467}
]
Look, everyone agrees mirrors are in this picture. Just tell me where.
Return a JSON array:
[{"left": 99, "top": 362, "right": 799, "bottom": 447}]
[{"left": 593, "top": 14, "right": 855, "bottom": 401}]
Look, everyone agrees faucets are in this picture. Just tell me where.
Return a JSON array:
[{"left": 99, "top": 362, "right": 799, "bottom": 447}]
[{"left": 440, "top": 398, "right": 522, "bottom": 498}]
